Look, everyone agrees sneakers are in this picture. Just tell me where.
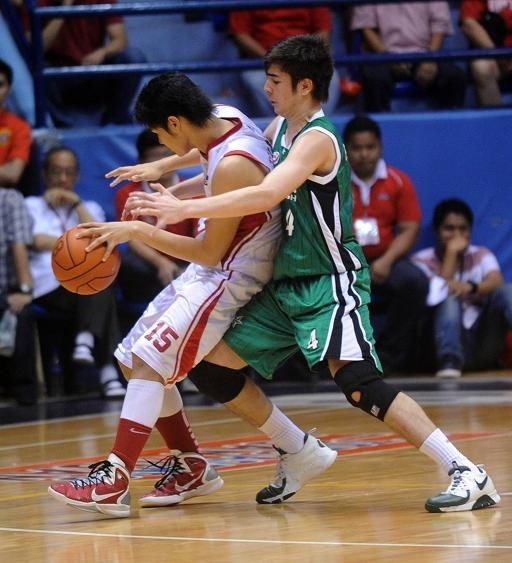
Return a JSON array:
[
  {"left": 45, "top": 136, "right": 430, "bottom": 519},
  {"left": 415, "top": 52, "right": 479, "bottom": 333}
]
[
  {"left": 99, "top": 375, "right": 127, "bottom": 399},
  {"left": 137, "top": 448, "right": 225, "bottom": 511},
  {"left": 48, "top": 450, "right": 134, "bottom": 518},
  {"left": 181, "top": 378, "right": 201, "bottom": 394},
  {"left": 435, "top": 357, "right": 464, "bottom": 380},
  {"left": 69, "top": 343, "right": 95, "bottom": 371},
  {"left": 255, "top": 431, "right": 339, "bottom": 504},
  {"left": 424, "top": 461, "right": 502, "bottom": 514}
]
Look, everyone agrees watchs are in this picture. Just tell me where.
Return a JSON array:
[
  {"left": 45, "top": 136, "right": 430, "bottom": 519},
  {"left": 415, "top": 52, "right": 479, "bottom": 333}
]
[
  {"left": 17, "top": 283, "right": 34, "bottom": 295},
  {"left": 467, "top": 279, "right": 479, "bottom": 295}
]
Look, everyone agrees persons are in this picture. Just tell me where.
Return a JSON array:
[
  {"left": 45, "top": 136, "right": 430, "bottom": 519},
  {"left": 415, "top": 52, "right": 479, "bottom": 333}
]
[
  {"left": 0, "top": 186, "right": 40, "bottom": 404},
  {"left": 23, "top": 145, "right": 129, "bottom": 397},
  {"left": 22, "top": 0, "right": 512, "bottom": 112},
  {"left": 114, "top": 127, "right": 207, "bottom": 323},
  {"left": 104, "top": 34, "right": 500, "bottom": 513},
  {"left": 340, "top": 116, "right": 429, "bottom": 379},
  {"left": 0, "top": 60, "right": 34, "bottom": 185},
  {"left": 412, "top": 198, "right": 512, "bottom": 378},
  {"left": 47, "top": 70, "right": 284, "bottom": 518}
]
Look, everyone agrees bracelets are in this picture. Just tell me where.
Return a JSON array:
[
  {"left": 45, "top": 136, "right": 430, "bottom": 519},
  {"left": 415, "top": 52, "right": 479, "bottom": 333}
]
[{"left": 72, "top": 198, "right": 82, "bottom": 208}]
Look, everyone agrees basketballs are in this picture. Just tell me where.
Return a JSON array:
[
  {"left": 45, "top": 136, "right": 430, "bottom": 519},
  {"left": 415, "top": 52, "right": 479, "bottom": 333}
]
[{"left": 50, "top": 225, "right": 119, "bottom": 295}]
